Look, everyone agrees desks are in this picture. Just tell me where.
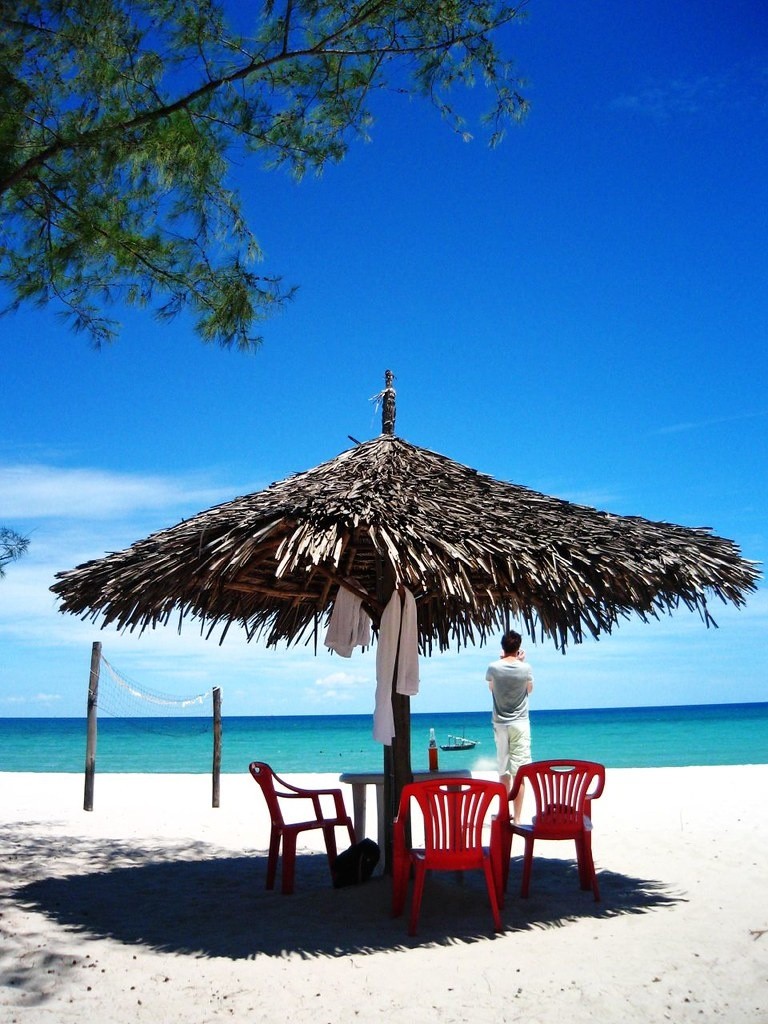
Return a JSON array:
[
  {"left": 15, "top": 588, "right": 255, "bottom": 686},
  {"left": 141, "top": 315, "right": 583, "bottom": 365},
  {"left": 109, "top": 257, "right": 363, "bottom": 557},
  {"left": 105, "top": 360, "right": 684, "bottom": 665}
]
[{"left": 339, "top": 768, "right": 470, "bottom": 880}]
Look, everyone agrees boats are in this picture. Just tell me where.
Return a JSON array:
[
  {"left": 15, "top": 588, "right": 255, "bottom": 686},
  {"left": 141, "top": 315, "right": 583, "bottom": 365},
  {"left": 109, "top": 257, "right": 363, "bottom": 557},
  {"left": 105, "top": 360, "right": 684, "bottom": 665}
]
[{"left": 439, "top": 735, "right": 477, "bottom": 751}]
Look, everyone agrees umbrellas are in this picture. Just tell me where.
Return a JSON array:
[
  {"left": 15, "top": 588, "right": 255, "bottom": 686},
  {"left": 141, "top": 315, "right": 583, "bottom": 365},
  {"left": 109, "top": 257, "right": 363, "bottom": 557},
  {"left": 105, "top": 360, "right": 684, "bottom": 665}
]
[{"left": 49, "top": 370, "right": 764, "bottom": 876}]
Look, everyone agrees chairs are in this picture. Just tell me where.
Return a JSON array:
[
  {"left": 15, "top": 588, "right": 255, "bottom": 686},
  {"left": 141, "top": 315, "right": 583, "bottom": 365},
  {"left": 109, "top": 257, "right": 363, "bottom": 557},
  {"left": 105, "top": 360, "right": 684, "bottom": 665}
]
[
  {"left": 491, "top": 760, "right": 605, "bottom": 904},
  {"left": 248, "top": 761, "right": 356, "bottom": 895},
  {"left": 392, "top": 780, "right": 510, "bottom": 937}
]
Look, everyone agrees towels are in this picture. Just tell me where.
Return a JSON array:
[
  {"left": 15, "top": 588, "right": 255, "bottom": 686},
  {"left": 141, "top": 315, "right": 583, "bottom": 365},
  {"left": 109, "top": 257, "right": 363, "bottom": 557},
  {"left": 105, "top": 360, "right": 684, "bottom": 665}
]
[
  {"left": 324, "top": 578, "right": 371, "bottom": 660},
  {"left": 373, "top": 585, "right": 419, "bottom": 746}
]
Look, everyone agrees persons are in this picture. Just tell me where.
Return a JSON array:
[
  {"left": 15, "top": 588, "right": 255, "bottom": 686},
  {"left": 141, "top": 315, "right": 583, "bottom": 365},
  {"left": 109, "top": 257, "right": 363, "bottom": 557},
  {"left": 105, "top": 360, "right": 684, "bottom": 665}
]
[{"left": 486, "top": 630, "right": 533, "bottom": 824}]
